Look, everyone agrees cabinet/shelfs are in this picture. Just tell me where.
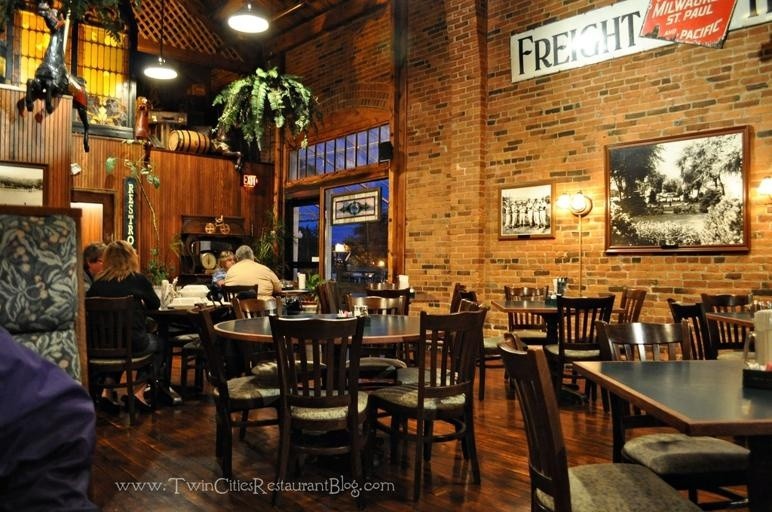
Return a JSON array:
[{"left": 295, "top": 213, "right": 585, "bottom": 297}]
[
  {"left": 153, "top": 281, "right": 178, "bottom": 311},
  {"left": 744, "top": 309, "right": 771, "bottom": 372},
  {"left": 753, "top": 298, "right": 771, "bottom": 315}
]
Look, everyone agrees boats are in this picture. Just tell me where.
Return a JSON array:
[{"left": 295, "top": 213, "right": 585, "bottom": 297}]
[{"left": 379, "top": 142, "right": 392, "bottom": 160}]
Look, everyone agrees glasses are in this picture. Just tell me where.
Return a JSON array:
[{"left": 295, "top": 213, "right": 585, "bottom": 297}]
[
  {"left": 243, "top": 174, "right": 258, "bottom": 188},
  {"left": 570, "top": 190, "right": 594, "bottom": 295},
  {"left": 758, "top": 174, "right": 772, "bottom": 194},
  {"left": 142, "top": 0, "right": 180, "bottom": 80},
  {"left": 226, "top": 0, "right": 270, "bottom": 35}
]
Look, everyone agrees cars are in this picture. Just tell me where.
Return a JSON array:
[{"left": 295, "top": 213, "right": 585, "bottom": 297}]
[
  {"left": 353, "top": 306, "right": 369, "bottom": 318},
  {"left": 277, "top": 279, "right": 294, "bottom": 289}
]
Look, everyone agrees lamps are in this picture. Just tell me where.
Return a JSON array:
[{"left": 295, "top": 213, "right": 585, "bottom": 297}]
[
  {"left": 570, "top": 190, "right": 594, "bottom": 295},
  {"left": 226, "top": 0, "right": 270, "bottom": 35},
  {"left": 142, "top": 0, "right": 180, "bottom": 80},
  {"left": 758, "top": 174, "right": 772, "bottom": 194},
  {"left": 243, "top": 174, "right": 258, "bottom": 188},
  {"left": 0, "top": 202, "right": 90, "bottom": 395}
]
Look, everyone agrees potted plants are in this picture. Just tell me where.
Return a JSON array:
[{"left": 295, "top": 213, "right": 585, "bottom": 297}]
[
  {"left": 330, "top": 186, "right": 382, "bottom": 226},
  {"left": 0, "top": 158, "right": 50, "bottom": 206},
  {"left": 603, "top": 124, "right": 751, "bottom": 258},
  {"left": 497, "top": 178, "right": 556, "bottom": 240}
]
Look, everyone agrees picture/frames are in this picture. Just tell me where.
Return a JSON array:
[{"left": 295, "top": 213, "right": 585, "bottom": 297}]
[
  {"left": 330, "top": 186, "right": 382, "bottom": 226},
  {"left": 603, "top": 124, "right": 751, "bottom": 258},
  {"left": 0, "top": 158, "right": 50, "bottom": 206},
  {"left": 497, "top": 178, "right": 556, "bottom": 240}
]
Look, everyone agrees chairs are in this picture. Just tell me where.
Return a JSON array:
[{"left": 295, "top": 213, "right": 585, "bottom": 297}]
[
  {"left": 0, "top": 202, "right": 90, "bottom": 395},
  {"left": 85, "top": 273, "right": 772, "bottom": 512}
]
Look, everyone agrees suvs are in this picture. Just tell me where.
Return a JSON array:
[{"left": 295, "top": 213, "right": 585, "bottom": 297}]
[{"left": 168, "top": 128, "right": 210, "bottom": 154}]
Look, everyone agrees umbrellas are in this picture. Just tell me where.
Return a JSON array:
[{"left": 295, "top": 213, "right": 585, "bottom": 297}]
[
  {"left": 100, "top": 394, "right": 121, "bottom": 411},
  {"left": 130, "top": 392, "right": 153, "bottom": 412}
]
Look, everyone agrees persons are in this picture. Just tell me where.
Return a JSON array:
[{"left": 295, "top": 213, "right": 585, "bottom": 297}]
[
  {"left": 83, "top": 243, "right": 109, "bottom": 292},
  {"left": 1, "top": 326, "right": 102, "bottom": 511},
  {"left": 84, "top": 239, "right": 161, "bottom": 415},
  {"left": 211, "top": 251, "right": 236, "bottom": 301},
  {"left": 503, "top": 196, "right": 551, "bottom": 229},
  {"left": 222, "top": 245, "right": 282, "bottom": 317}
]
